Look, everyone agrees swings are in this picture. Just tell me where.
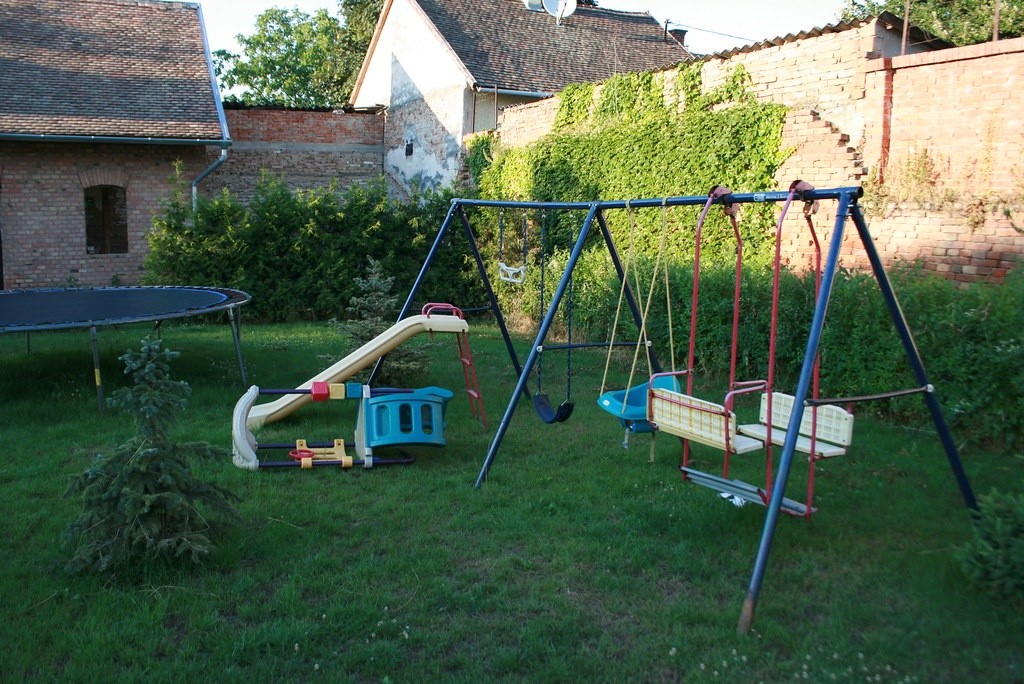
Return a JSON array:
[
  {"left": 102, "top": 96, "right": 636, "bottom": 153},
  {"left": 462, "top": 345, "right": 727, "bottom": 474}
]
[{"left": 532, "top": 188, "right": 854, "bottom": 520}]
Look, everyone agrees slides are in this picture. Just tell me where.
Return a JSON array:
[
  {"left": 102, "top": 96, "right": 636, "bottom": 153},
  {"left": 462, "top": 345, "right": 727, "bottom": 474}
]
[{"left": 244, "top": 313, "right": 471, "bottom": 432}]
